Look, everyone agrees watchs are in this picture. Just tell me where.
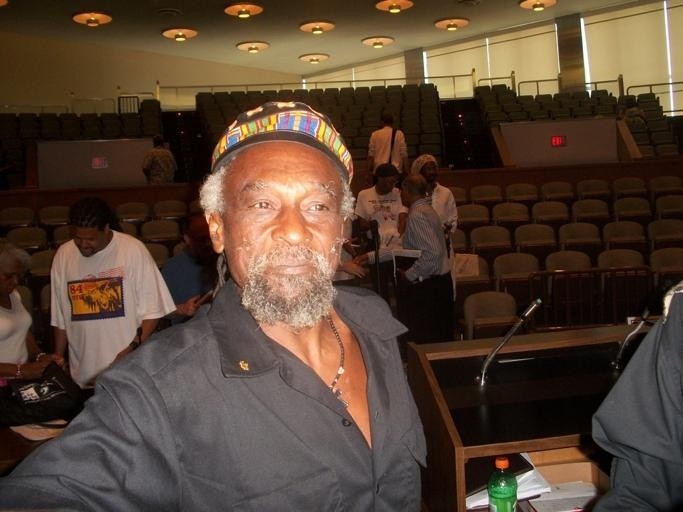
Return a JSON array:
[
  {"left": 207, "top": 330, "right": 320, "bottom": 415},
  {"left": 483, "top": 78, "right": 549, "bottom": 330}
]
[{"left": 14, "top": 362, "right": 23, "bottom": 380}]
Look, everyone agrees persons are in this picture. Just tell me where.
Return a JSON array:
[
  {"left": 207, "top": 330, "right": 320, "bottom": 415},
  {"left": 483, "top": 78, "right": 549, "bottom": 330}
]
[
  {"left": 142, "top": 135, "right": 176, "bottom": 185},
  {"left": 0, "top": 241, "right": 62, "bottom": 381},
  {"left": 362, "top": 176, "right": 457, "bottom": 344},
  {"left": 618, "top": 97, "right": 648, "bottom": 130},
  {"left": 354, "top": 163, "right": 407, "bottom": 255},
  {"left": 51, "top": 198, "right": 175, "bottom": 389},
  {"left": 321, "top": 232, "right": 368, "bottom": 281},
  {"left": 408, "top": 154, "right": 459, "bottom": 273},
  {"left": 1, "top": 102, "right": 428, "bottom": 510},
  {"left": 161, "top": 212, "right": 230, "bottom": 322},
  {"left": 587, "top": 280, "right": 682, "bottom": 511},
  {"left": 362, "top": 114, "right": 409, "bottom": 181}
]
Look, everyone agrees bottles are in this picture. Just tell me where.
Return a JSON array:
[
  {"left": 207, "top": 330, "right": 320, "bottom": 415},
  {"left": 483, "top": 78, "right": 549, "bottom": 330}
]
[{"left": 486, "top": 457, "right": 518, "bottom": 512}]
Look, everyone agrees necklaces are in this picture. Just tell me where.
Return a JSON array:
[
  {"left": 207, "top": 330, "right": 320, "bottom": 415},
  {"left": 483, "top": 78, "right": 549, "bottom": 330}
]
[{"left": 300, "top": 305, "right": 360, "bottom": 415}]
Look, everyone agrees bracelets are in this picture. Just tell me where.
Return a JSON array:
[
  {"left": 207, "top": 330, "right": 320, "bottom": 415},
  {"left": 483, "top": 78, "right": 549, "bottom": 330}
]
[
  {"left": 129, "top": 340, "right": 140, "bottom": 350},
  {"left": 34, "top": 349, "right": 46, "bottom": 362}
]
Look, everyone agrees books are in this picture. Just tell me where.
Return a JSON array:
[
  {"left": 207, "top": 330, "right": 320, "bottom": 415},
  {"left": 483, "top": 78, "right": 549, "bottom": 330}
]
[
  {"left": 524, "top": 480, "right": 599, "bottom": 511},
  {"left": 458, "top": 446, "right": 554, "bottom": 509}
]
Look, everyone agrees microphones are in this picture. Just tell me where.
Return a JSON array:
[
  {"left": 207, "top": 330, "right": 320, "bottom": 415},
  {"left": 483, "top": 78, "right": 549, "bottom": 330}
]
[
  {"left": 369, "top": 219, "right": 380, "bottom": 257},
  {"left": 609, "top": 272, "right": 673, "bottom": 372},
  {"left": 473, "top": 295, "right": 545, "bottom": 393}
]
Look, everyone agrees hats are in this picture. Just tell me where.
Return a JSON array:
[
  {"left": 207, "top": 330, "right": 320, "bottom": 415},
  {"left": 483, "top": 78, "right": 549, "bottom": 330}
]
[{"left": 209, "top": 99, "right": 356, "bottom": 188}]
